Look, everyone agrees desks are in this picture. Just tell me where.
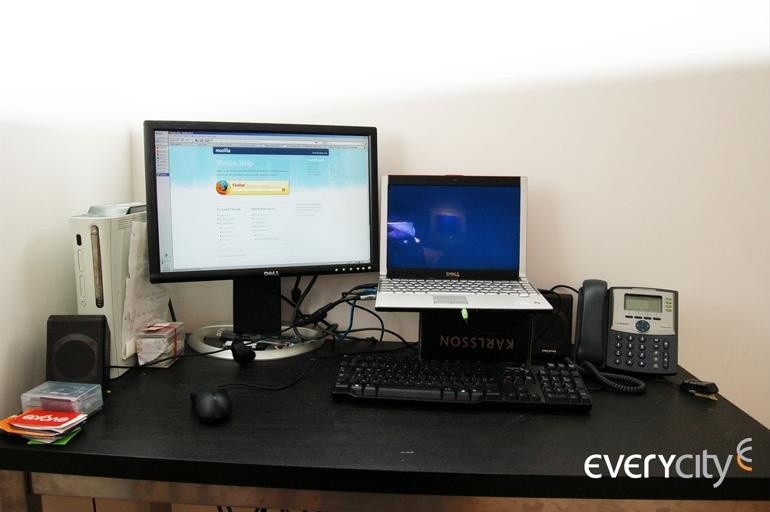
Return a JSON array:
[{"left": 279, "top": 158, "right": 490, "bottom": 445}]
[{"left": 1, "top": 331, "right": 770, "bottom": 512}]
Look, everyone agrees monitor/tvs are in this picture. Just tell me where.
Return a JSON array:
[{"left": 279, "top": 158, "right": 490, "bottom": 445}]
[{"left": 143, "top": 118, "right": 378, "bottom": 362}]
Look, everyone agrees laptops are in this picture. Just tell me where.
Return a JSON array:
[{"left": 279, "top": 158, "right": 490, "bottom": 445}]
[{"left": 374, "top": 172, "right": 556, "bottom": 317}]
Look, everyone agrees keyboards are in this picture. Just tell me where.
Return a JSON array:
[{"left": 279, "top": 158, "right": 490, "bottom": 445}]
[{"left": 331, "top": 347, "right": 595, "bottom": 413}]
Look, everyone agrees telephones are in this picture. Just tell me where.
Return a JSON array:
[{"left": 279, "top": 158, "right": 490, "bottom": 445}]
[{"left": 574, "top": 279, "right": 678, "bottom": 392}]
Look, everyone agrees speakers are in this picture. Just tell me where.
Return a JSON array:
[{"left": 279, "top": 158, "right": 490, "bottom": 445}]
[
  {"left": 46, "top": 313, "right": 114, "bottom": 398},
  {"left": 418, "top": 288, "right": 573, "bottom": 366}
]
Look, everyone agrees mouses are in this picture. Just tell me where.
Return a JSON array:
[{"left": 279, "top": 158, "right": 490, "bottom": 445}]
[{"left": 189, "top": 386, "right": 233, "bottom": 426}]
